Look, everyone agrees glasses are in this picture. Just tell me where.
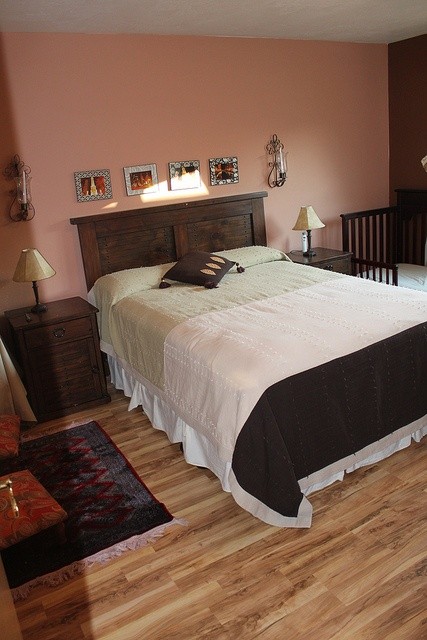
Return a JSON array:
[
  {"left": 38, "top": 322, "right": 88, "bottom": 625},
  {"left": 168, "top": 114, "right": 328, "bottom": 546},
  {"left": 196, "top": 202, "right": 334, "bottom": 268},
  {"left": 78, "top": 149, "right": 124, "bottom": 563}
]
[
  {"left": 2, "top": 154, "right": 35, "bottom": 223},
  {"left": 13, "top": 247, "right": 57, "bottom": 311},
  {"left": 291, "top": 205, "right": 326, "bottom": 256},
  {"left": 266, "top": 134, "right": 289, "bottom": 188}
]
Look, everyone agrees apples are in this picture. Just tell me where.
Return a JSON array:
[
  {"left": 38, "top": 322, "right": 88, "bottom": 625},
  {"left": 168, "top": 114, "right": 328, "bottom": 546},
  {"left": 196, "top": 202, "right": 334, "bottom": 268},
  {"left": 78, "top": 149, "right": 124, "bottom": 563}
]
[
  {"left": 21, "top": 170, "right": 27, "bottom": 203},
  {"left": 279, "top": 147, "right": 284, "bottom": 173}
]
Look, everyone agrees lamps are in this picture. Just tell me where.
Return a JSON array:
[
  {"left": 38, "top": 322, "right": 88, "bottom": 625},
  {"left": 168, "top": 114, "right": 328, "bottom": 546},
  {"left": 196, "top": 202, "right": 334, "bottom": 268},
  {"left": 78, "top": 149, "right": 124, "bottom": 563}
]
[{"left": 340, "top": 204, "right": 427, "bottom": 287}]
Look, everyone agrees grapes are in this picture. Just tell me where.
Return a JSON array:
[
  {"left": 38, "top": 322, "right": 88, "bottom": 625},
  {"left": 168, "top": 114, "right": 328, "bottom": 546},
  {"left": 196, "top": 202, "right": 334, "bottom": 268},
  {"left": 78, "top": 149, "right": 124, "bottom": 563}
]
[
  {"left": 4, "top": 296, "right": 110, "bottom": 421},
  {"left": 285, "top": 247, "right": 354, "bottom": 275}
]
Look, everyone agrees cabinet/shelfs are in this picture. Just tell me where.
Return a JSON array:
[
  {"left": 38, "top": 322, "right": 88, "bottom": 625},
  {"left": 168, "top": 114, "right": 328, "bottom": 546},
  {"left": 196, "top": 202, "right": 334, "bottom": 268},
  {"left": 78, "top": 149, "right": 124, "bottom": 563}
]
[{"left": 301, "top": 232, "right": 307, "bottom": 252}]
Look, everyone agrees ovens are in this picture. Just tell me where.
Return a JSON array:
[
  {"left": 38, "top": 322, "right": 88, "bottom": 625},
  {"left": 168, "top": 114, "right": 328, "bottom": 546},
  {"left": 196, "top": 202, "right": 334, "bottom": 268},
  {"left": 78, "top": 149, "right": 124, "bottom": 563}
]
[{"left": 70, "top": 191, "right": 427, "bottom": 530}]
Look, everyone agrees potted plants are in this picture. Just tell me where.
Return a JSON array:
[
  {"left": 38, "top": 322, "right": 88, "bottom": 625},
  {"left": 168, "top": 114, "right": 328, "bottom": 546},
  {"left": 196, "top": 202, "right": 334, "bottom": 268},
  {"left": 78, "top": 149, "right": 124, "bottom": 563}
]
[
  {"left": 209, "top": 157, "right": 239, "bottom": 185},
  {"left": 168, "top": 160, "right": 200, "bottom": 190},
  {"left": 74, "top": 169, "right": 113, "bottom": 202},
  {"left": 123, "top": 163, "right": 160, "bottom": 196}
]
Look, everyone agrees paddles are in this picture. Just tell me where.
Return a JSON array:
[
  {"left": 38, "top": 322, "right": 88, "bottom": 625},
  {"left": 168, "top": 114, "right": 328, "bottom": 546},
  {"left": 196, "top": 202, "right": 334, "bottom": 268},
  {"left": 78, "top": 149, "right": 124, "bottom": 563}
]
[{"left": 1, "top": 421, "right": 191, "bottom": 602}]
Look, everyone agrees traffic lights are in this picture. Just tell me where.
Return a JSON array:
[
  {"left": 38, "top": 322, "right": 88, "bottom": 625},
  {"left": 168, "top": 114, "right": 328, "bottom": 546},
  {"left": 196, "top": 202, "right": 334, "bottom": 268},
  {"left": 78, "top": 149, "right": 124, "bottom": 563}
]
[
  {"left": 0, "top": 413, "right": 22, "bottom": 459},
  {"left": 159, "top": 250, "right": 245, "bottom": 288},
  {"left": 0, "top": 469, "right": 69, "bottom": 552}
]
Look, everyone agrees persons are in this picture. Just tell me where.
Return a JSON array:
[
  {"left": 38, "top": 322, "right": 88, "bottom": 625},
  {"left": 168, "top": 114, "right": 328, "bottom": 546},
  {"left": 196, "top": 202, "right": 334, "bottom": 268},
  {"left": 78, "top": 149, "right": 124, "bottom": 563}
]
[{"left": 131, "top": 174, "right": 146, "bottom": 189}]
